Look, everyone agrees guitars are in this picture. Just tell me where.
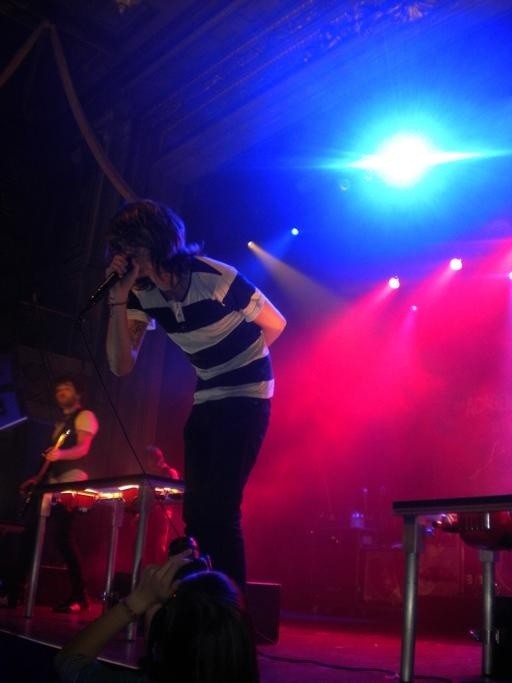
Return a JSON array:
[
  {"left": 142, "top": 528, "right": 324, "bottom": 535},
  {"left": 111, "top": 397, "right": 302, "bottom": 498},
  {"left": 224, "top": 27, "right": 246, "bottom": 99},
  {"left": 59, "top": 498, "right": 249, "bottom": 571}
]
[{"left": 17, "top": 428, "right": 72, "bottom": 517}]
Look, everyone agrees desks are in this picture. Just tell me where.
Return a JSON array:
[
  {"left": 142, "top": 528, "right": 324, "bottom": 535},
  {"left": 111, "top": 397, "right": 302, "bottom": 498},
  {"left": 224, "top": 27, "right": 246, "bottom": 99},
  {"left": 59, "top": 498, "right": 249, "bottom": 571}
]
[
  {"left": 392, "top": 495, "right": 512, "bottom": 682},
  {"left": 23, "top": 473, "right": 184, "bottom": 640}
]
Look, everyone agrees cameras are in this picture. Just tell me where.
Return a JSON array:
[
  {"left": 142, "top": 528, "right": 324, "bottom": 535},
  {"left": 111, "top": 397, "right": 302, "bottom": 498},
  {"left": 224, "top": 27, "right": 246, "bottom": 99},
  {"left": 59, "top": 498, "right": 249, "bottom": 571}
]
[{"left": 168, "top": 537, "right": 212, "bottom": 580}]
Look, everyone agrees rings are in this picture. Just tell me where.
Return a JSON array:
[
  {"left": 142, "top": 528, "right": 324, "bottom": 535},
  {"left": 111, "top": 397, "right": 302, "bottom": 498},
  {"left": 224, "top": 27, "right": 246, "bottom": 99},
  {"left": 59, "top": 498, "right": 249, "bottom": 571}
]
[
  {"left": 158, "top": 568, "right": 166, "bottom": 574},
  {"left": 150, "top": 565, "right": 159, "bottom": 570}
]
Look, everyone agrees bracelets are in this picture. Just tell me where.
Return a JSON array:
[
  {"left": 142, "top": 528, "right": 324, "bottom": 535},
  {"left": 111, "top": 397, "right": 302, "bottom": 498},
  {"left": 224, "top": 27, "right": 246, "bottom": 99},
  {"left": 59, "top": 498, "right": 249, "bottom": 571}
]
[
  {"left": 108, "top": 300, "right": 129, "bottom": 307},
  {"left": 121, "top": 597, "right": 136, "bottom": 622}
]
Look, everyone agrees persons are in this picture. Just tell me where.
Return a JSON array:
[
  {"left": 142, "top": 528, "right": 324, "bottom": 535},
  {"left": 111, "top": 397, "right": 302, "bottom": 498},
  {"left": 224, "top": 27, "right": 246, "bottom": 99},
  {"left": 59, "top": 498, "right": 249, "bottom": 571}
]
[
  {"left": 104, "top": 197, "right": 288, "bottom": 593},
  {"left": 17, "top": 377, "right": 102, "bottom": 611},
  {"left": 49, "top": 548, "right": 262, "bottom": 682}
]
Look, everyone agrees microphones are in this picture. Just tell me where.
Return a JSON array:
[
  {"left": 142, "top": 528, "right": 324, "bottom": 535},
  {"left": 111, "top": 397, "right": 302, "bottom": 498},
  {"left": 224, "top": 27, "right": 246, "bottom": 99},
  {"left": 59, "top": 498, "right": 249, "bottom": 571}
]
[{"left": 87, "top": 254, "right": 139, "bottom": 308}]
